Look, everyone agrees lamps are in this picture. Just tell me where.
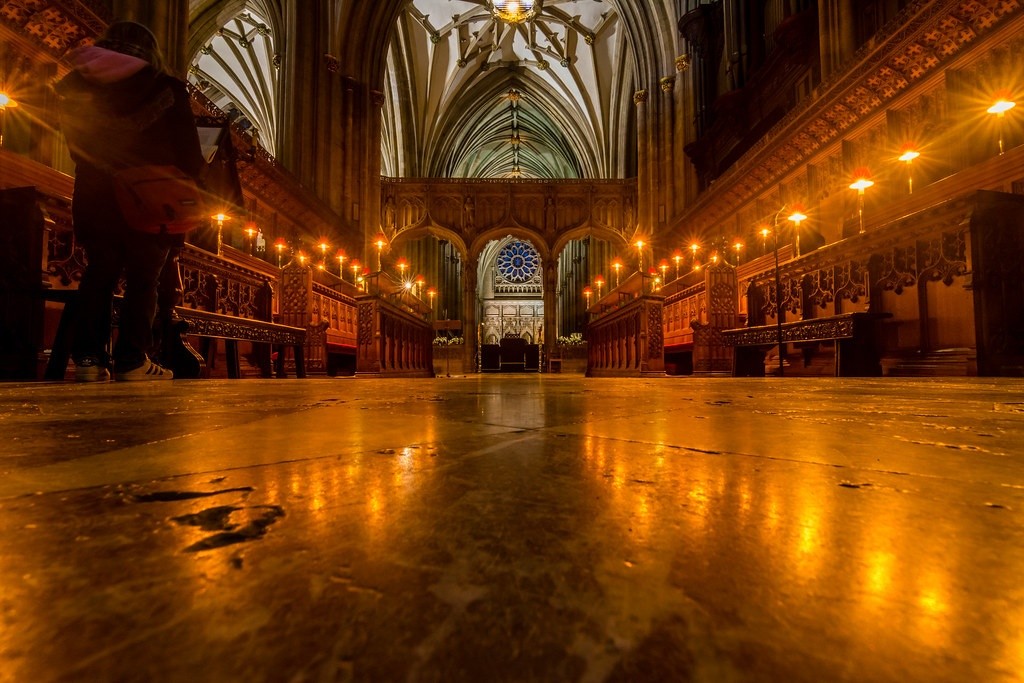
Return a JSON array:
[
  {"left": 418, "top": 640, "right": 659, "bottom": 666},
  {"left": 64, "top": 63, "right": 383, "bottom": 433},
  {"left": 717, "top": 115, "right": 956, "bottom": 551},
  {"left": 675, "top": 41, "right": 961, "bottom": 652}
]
[
  {"left": 849, "top": 168, "right": 875, "bottom": 233},
  {"left": 318, "top": 237, "right": 330, "bottom": 270},
  {"left": 211, "top": 205, "right": 231, "bottom": 257},
  {"left": 610, "top": 236, "right": 646, "bottom": 286},
  {"left": 671, "top": 249, "right": 684, "bottom": 279},
  {"left": 374, "top": 232, "right": 387, "bottom": 272},
  {"left": 396, "top": 256, "right": 408, "bottom": 287},
  {"left": 427, "top": 286, "right": 436, "bottom": 309},
  {"left": 658, "top": 258, "right": 669, "bottom": 286},
  {"left": 244, "top": 221, "right": 259, "bottom": 255},
  {"left": 273, "top": 237, "right": 289, "bottom": 268},
  {"left": 415, "top": 274, "right": 426, "bottom": 299},
  {"left": 583, "top": 287, "right": 594, "bottom": 309},
  {"left": 594, "top": 275, "right": 605, "bottom": 300},
  {"left": 336, "top": 248, "right": 347, "bottom": 279},
  {"left": 985, "top": 86, "right": 1016, "bottom": 154},
  {"left": 688, "top": 237, "right": 701, "bottom": 271},
  {"left": 732, "top": 204, "right": 808, "bottom": 267},
  {"left": 349, "top": 259, "right": 361, "bottom": 286},
  {"left": 898, "top": 142, "right": 920, "bottom": 194}
]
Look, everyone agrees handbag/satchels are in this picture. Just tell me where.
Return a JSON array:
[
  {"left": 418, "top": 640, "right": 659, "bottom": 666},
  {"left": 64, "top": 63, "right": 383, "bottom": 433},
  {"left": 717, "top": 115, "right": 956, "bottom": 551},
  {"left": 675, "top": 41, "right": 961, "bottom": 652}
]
[{"left": 112, "top": 161, "right": 213, "bottom": 238}]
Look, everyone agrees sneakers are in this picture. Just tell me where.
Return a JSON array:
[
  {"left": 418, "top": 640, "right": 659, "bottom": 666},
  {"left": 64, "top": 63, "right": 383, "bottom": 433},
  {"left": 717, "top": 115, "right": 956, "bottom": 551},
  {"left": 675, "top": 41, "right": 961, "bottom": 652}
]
[
  {"left": 74, "top": 355, "right": 111, "bottom": 383},
  {"left": 115, "top": 354, "right": 174, "bottom": 380}
]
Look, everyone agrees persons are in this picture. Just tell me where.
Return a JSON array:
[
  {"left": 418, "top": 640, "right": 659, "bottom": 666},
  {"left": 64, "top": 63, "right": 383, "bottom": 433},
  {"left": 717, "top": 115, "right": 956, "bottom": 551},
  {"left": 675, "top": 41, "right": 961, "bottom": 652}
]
[{"left": 55, "top": 21, "right": 202, "bottom": 382}]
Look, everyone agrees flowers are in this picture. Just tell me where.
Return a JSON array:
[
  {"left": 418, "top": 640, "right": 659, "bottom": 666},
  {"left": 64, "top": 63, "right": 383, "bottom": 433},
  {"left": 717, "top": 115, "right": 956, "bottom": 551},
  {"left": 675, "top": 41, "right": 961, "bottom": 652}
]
[
  {"left": 558, "top": 332, "right": 588, "bottom": 347},
  {"left": 432, "top": 336, "right": 464, "bottom": 346}
]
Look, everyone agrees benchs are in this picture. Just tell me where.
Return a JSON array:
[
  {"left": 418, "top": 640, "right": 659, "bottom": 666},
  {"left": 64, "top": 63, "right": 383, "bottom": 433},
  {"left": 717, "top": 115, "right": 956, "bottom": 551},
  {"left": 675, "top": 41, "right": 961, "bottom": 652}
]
[
  {"left": 215, "top": 243, "right": 359, "bottom": 356},
  {"left": 721, "top": 312, "right": 894, "bottom": 378},
  {"left": 0, "top": 185, "right": 307, "bottom": 383},
  {"left": 663, "top": 257, "right": 740, "bottom": 378}
]
